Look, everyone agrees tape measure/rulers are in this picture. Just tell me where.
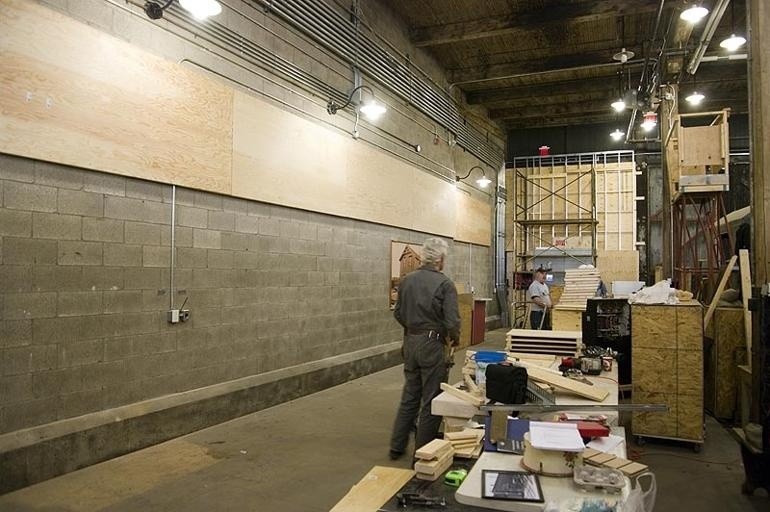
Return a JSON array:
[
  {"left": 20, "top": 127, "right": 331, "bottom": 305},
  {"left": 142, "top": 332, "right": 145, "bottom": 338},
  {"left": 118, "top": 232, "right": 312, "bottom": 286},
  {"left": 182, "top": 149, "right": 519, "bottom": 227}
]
[{"left": 445, "top": 468, "right": 467, "bottom": 486}]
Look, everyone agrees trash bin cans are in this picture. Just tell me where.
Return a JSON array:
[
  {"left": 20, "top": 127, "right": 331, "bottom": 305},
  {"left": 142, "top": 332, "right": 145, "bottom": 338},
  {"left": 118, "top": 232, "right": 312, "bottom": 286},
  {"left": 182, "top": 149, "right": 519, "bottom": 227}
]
[{"left": 582, "top": 299, "right": 631, "bottom": 398}]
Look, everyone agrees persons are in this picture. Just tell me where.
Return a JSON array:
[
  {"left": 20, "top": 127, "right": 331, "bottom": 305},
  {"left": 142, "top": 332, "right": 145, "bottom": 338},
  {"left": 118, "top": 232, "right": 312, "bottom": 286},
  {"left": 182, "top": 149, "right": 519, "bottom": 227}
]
[
  {"left": 527, "top": 267, "right": 553, "bottom": 330},
  {"left": 389, "top": 237, "right": 462, "bottom": 466}
]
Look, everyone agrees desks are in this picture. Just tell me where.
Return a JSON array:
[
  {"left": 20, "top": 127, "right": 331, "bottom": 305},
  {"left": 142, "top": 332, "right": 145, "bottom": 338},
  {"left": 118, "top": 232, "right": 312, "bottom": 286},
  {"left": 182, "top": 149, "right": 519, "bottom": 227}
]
[
  {"left": 431, "top": 351, "right": 618, "bottom": 426},
  {"left": 472, "top": 297, "right": 493, "bottom": 344},
  {"left": 327, "top": 425, "right": 627, "bottom": 512}
]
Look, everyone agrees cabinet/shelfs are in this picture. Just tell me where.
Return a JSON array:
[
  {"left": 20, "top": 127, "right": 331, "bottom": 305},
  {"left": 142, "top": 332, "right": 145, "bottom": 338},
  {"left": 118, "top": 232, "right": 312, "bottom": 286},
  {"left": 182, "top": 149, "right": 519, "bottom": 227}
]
[
  {"left": 704, "top": 307, "right": 747, "bottom": 419},
  {"left": 630, "top": 298, "right": 707, "bottom": 454}
]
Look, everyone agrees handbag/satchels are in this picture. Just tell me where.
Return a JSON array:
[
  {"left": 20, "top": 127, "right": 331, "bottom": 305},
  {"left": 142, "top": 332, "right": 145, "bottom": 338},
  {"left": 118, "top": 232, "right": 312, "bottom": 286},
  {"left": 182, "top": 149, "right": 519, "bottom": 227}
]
[{"left": 483, "top": 362, "right": 529, "bottom": 405}]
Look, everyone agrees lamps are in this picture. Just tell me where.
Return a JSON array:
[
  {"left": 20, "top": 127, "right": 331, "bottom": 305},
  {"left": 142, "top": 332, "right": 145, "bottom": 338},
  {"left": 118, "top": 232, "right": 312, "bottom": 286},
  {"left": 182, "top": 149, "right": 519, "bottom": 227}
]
[
  {"left": 609, "top": 67, "right": 629, "bottom": 115},
  {"left": 141, "top": 0, "right": 221, "bottom": 18},
  {"left": 325, "top": 84, "right": 387, "bottom": 123},
  {"left": 455, "top": 165, "right": 492, "bottom": 188},
  {"left": 680, "top": 0, "right": 710, "bottom": 25},
  {"left": 609, "top": 115, "right": 626, "bottom": 144},
  {"left": 720, "top": 1, "right": 746, "bottom": 52},
  {"left": 612, "top": 14, "right": 635, "bottom": 64},
  {"left": 684, "top": 72, "right": 706, "bottom": 107}
]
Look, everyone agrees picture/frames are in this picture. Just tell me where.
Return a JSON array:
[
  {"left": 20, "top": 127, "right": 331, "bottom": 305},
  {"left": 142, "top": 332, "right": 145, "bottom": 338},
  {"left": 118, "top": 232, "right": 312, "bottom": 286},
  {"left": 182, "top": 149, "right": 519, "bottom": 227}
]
[{"left": 390, "top": 242, "right": 424, "bottom": 312}]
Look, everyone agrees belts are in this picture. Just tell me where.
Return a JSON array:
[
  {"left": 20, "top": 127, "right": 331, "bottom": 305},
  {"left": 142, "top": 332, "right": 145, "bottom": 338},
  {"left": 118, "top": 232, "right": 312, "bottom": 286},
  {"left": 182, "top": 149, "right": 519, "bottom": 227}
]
[{"left": 404, "top": 326, "right": 448, "bottom": 344}]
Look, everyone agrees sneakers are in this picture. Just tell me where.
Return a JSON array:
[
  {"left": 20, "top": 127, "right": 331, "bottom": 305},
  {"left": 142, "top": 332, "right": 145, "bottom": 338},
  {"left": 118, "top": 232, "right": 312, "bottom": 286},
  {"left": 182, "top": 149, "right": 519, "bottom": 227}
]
[{"left": 389, "top": 445, "right": 422, "bottom": 470}]
